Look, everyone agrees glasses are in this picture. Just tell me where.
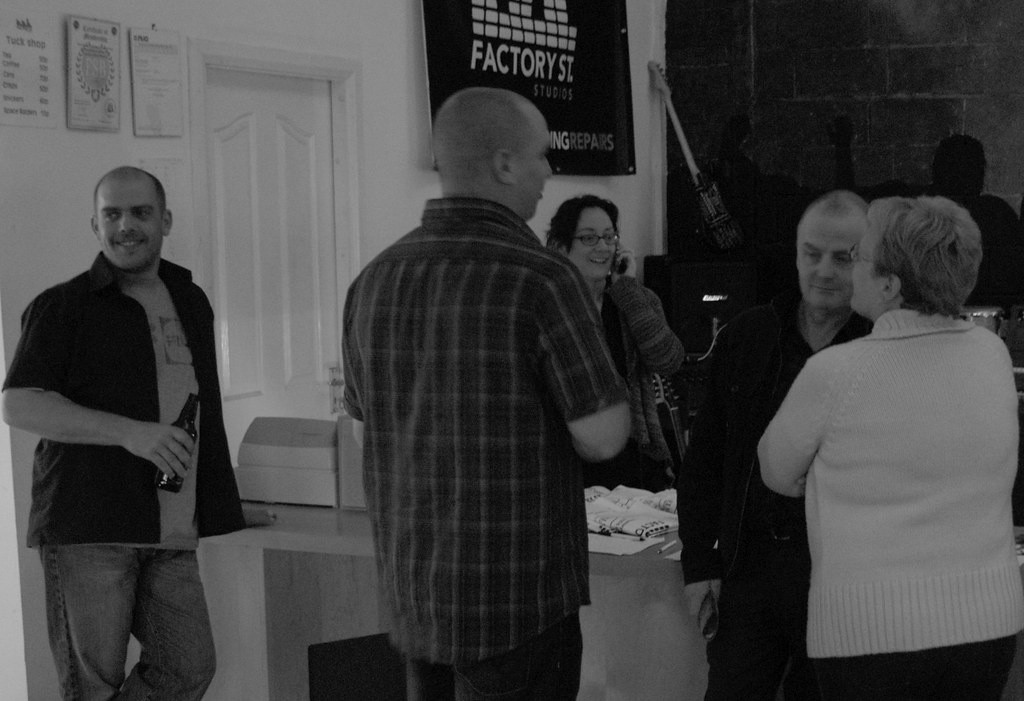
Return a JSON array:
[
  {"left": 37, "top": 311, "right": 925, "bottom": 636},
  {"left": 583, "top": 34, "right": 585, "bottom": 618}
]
[{"left": 575, "top": 233, "right": 620, "bottom": 246}]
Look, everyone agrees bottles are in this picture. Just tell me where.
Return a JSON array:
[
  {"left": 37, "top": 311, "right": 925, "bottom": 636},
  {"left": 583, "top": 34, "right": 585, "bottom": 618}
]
[{"left": 154, "top": 392, "right": 199, "bottom": 493}]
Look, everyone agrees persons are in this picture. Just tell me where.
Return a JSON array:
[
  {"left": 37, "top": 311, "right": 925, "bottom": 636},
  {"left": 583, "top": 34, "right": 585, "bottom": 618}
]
[
  {"left": 0, "top": 165, "right": 248, "bottom": 701},
  {"left": 342, "top": 88, "right": 632, "bottom": 701},
  {"left": 544, "top": 133, "right": 1024, "bottom": 701}
]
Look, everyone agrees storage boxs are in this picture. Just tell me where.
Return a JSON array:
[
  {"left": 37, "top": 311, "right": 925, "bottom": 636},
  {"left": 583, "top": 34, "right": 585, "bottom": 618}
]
[{"left": 646, "top": 254, "right": 758, "bottom": 351}]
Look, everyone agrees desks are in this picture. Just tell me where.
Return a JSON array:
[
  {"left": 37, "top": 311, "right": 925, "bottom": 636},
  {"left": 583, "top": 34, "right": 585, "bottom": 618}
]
[{"left": 196, "top": 498, "right": 708, "bottom": 701}]
